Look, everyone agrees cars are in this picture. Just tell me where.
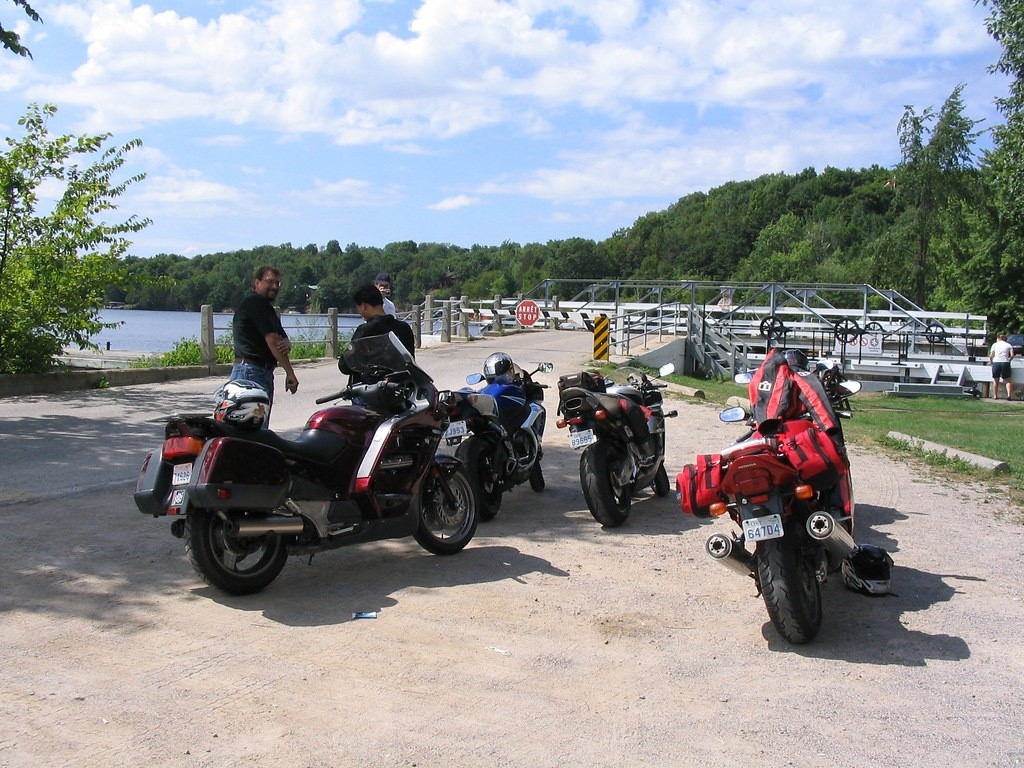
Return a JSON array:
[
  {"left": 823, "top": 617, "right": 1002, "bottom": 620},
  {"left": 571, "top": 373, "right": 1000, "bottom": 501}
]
[{"left": 1007, "top": 333, "right": 1024, "bottom": 358}]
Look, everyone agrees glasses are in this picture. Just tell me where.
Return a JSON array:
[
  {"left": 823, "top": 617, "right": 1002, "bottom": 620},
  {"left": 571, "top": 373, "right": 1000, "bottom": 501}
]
[{"left": 260, "top": 279, "right": 282, "bottom": 287}]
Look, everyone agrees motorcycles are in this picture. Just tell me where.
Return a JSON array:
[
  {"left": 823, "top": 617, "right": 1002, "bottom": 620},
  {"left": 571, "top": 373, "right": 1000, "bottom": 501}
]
[
  {"left": 674, "top": 346, "right": 858, "bottom": 645},
  {"left": 556, "top": 367, "right": 679, "bottom": 530},
  {"left": 132, "top": 331, "right": 483, "bottom": 596},
  {"left": 437, "top": 350, "right": 552, "bottom": 521}
]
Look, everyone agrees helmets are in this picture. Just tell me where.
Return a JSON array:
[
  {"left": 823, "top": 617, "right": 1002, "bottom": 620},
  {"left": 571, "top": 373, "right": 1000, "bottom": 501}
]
[
  {"left": 484, "top": 352, "right": 515, "bottom": 383},
  {"left": 841, "top": 544, "right": 892, "bottom": 596},
  {"left": 783, "top": 350, "right": 807, "bottom": 371},
  {"left": 215, "top": 378, "right": 270, "bottom": 427}
]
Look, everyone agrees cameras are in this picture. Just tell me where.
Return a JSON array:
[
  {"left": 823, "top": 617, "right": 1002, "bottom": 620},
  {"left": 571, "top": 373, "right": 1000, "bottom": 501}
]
[{"left": 382, "top": 289, "right": 391, "bottom": 296}]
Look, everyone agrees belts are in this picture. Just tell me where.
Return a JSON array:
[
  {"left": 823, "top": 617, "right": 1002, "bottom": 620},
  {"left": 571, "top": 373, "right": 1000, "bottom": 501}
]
[{"left": 235, "top": 357, "right": 274, "bottom": 370}]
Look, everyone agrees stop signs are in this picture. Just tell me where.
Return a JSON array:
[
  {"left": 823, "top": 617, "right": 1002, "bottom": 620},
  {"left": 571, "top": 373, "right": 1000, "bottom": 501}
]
[{"left": 514, "top": 300, "right": 540, "bottom": 327}]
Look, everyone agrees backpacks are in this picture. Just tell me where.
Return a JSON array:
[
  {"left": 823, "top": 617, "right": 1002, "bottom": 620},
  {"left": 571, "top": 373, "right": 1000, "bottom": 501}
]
[{"left": 558, "top": 372, "right": 600, "bottom": 392}]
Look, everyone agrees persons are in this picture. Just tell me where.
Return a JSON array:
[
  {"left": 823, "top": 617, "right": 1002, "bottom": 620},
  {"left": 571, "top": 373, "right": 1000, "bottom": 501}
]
[
  {"left": 338, "top": 284, "right": 415, "bottom": 406},
  {"left": 363, "top": 273, "right": 397, "bottom": 323},
  {"left": 717, "top": 293, "right": 732, "bottom": 326},
  {"left": 988, "top": 334, "right": 1014, "bottom": 400},
  {"left": 229, "top": 266, "right": 299, "bottom": 429}
]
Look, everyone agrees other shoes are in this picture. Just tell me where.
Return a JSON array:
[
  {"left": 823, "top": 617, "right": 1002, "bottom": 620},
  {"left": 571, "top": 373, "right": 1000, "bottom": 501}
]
[
  {"left": 1007, "top": 397, "right": 1011, "bottom": 400},
  {"left": 994, "top": 396, "right": 997, "bottom": 399}
]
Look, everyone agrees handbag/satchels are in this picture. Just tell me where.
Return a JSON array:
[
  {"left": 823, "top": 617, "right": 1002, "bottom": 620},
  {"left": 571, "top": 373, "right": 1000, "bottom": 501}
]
[
  {"left": 778, "top": 427, "right": 847, "bottom": 492},
  {"left": 676, "top": 453, "right": 725, "bottom": 513}
]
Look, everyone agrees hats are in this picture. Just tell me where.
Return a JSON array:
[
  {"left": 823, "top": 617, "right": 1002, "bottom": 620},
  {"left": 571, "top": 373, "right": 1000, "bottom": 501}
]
[{"left": 374, "top": 271, "right": 391, "bottom": 286}]
